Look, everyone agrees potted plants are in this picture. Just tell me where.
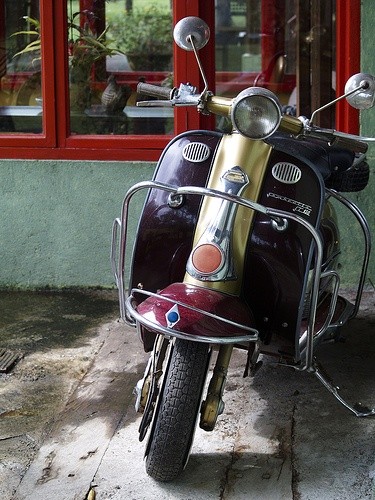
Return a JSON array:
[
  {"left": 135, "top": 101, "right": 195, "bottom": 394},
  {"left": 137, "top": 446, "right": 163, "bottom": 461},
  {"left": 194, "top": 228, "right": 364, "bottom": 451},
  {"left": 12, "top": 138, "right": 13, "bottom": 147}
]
[{"left": 9, "top": 12, "right": 128, "bottom": 112}]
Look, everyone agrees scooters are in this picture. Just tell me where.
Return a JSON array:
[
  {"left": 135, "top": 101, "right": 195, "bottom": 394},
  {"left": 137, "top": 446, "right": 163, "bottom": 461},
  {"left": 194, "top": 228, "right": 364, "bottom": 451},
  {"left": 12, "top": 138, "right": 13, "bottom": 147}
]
[{"left": 101, "top": 15, "right": 374, "bottom": 481}]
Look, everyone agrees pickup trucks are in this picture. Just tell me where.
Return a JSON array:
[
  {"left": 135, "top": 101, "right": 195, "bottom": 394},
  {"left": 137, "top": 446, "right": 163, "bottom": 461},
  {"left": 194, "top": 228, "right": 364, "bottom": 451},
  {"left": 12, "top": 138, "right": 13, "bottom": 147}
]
[{"left": 2, "top": 39, "right": 298, "bottom": 110}]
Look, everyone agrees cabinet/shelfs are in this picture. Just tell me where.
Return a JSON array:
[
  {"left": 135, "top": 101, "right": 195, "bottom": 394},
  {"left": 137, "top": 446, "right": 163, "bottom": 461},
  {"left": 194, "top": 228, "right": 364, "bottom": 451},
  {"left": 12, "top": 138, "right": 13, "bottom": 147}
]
[{"left": 0, "top": 105, "right": 174, "bottom": 135}]
